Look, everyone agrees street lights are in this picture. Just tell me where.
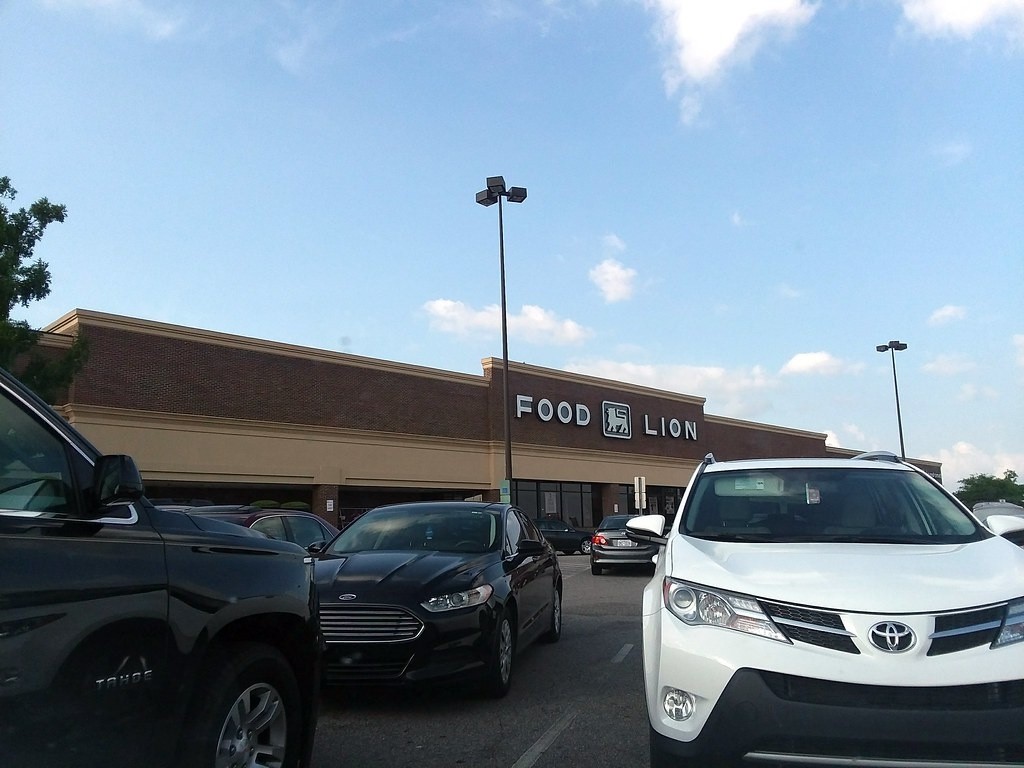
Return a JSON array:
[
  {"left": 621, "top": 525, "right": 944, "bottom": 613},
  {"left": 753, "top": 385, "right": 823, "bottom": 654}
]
[
  {"left": 473, "top": 175, "right": 528, "bottom": 505},
  {"left": 875, "top": 339, "right": 908, "bottom": 462}
]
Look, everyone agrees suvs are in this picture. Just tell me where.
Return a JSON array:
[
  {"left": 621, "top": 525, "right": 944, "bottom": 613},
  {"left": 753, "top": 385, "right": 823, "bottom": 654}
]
[
  {"left": 0, "top": 361, "right": 324, "bottom": 767},
  {"left": 625, "top": 450, "right": 1024, "bottom": 768}
]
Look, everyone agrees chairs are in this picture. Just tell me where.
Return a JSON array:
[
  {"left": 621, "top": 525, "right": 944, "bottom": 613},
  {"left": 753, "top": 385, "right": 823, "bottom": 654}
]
[
  {"left": 824, "top": 494, "right": 878, "bottom": 536},
  {"left": 705, "top": 494, "right": 771, "bottom": 535}
]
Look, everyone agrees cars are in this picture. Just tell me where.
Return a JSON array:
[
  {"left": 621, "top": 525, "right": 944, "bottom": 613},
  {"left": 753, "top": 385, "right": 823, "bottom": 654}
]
[
  {"left": 589, "top": 515, "right": 671, "bottom": 575},
  {"left": 306, "top": 500, "right": 565, "bottom": 699},
  {"left": 531, "top": 519, "right": 596, "bottom": 556},
  {"left": 153, "top": 502, "right": 342, "bottom": 554}
]
